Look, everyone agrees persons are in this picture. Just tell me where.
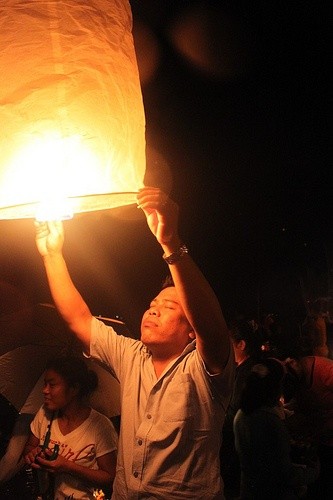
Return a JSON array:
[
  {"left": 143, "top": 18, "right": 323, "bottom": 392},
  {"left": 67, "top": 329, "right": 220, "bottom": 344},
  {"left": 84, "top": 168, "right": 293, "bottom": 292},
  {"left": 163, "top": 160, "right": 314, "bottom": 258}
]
[
  {"left": 30, "top": 184, "right": 238, "bottom": 500},
  {"left": 223, "top": 287, "right": 333, "bottom": 500},
  {"left": 24, "top": 355, "right": 119, "bottom": 500},
  {"left": 0, "top": 330, "right": 124, "bottom": 490}
]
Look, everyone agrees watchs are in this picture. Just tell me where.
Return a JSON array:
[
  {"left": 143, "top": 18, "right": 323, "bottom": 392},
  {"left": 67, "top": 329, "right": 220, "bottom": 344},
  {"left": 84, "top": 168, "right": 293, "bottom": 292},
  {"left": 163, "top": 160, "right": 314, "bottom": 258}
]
[{"left": 161, "top": 243, "right": 189, "bottom": 265}]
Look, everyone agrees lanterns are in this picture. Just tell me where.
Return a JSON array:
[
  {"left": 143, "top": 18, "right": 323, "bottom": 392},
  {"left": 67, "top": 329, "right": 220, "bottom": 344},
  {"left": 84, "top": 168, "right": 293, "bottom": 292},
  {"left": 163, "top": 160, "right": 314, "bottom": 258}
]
[{"left": 0, "top": 0, "right": 149, "bottom": 221}]
